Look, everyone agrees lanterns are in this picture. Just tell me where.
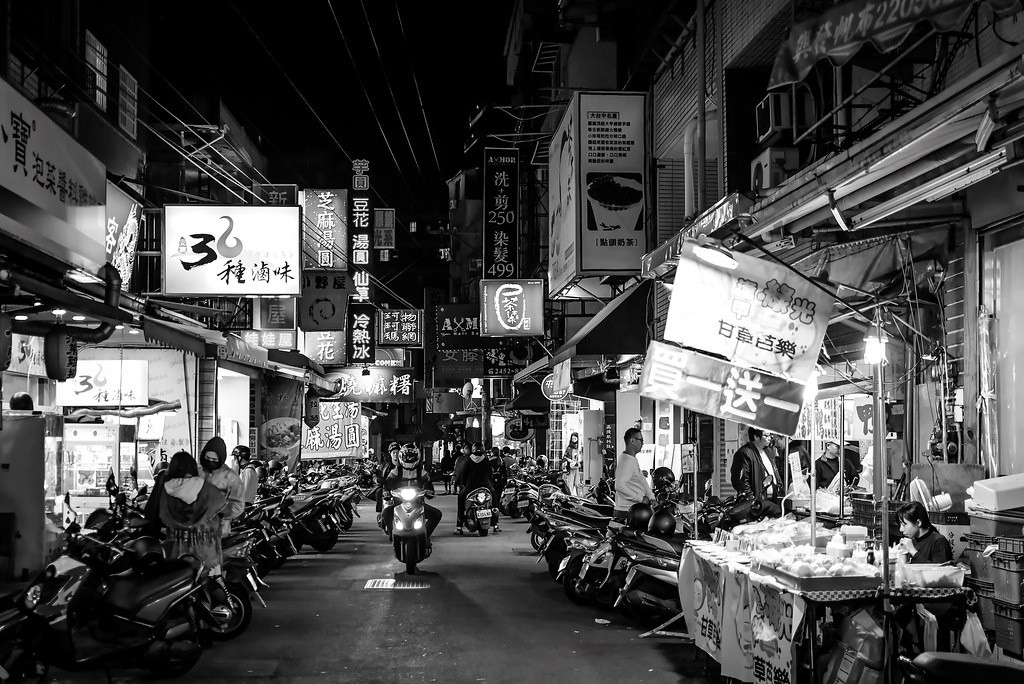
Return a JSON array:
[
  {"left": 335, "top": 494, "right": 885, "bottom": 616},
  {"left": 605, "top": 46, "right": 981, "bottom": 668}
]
[
  {"left": 462, "top": 381, "right": 473, "bottom": 398},
  {"left": 44, "top": 311, "right": 78, "bottom": 383},
  {"left": 0, "top": 313, "right": 12, "bottom": 371},
  {"left": 304, "top": 389, "right": 320, "bottom": 433}
]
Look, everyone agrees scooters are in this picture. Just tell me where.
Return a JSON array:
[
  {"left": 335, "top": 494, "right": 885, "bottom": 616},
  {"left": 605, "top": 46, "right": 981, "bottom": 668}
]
[
  {"left": 374, "top": 474, "right": 440, "bottom": 575},
  {"left": 0, "top": 460, "right": 382, "bottom": 680},
  {"left": 464, "top": 485, "right": 501, "bottom": 540},
  {"left": 499, "top": 459, "right": 785, "bottom": 634}
]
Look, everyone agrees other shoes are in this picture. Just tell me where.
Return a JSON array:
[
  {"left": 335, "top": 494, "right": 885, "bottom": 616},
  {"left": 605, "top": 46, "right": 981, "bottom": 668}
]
[
  {"left": 448, "top": 490, "right": 451, "bottom": 494},
  {"left": 494, "top": 526, "right": 503, "bottom": 532},
  {"left": 453, "top": 530, "right": 463, "bottom": 535}
]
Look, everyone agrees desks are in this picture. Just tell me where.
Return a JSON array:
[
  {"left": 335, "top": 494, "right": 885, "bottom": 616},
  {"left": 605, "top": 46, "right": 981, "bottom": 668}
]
[{"left": 677, "top": 544, "right": 979, "bottom": 684}]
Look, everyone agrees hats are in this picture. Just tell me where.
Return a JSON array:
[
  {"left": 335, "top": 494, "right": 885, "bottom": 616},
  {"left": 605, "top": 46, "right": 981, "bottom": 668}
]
[
  {"left": 824, "top": 439, "right": 850, "bottom": 447},
  {"left": 200, "top": 437, "right": 227, "bottom": 468}
]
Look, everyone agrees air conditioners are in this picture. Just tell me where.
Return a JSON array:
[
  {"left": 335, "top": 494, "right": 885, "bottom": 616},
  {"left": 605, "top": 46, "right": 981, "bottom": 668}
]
[
  {"left": 750, "top": 147, "right": 799, "bottom": 195},
  {"left": 449, "top": 199, "right": 457, "bottom": 210},
  {"left": 755, "top": 92, "right": 791, "bottom": 147}
]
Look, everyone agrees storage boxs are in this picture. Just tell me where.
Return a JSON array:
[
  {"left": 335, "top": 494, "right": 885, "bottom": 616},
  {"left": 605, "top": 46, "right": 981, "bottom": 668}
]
[
  {"left": 850, "top": 498, "right": 970, "bottom": 540},
  {"left": 901, "top": 564, "right": 965, "bottom": 588},
  {"left": 960, "top": 473, "right": 1024, "bottom": 654}
]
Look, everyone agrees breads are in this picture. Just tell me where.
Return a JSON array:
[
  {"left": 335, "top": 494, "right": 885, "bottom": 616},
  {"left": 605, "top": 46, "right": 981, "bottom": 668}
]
[{"left": 753, "top": 542, "right": 859, "bottom": 576}]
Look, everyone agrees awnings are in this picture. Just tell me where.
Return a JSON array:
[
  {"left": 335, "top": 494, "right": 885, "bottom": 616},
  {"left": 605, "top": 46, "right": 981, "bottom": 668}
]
[
  {"left": 0, "top": 232, "right": 337, "bottom": 398},
  {"left": 514, "top": 44, "right": 1024, "bottom": 382}
]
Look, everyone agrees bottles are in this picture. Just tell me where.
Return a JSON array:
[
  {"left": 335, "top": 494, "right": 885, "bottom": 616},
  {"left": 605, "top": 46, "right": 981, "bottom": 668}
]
[
  {"left": 867, "top": 546, "right": 875, "bottom": 565},
  {"left": 832, "top": 529, "right": 843, "bottom": 545}
]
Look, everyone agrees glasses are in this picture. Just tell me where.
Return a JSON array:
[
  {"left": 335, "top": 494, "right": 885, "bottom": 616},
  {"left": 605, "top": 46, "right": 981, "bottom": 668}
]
[
  {"left": 633, "top": 436, "right": 643, "bottom": 444},
  {"left": 775, "top": 438, "right": 781, "bottom": 443},
  {"left": 762, "top": 434, "right": 770, "bottom": 438}
]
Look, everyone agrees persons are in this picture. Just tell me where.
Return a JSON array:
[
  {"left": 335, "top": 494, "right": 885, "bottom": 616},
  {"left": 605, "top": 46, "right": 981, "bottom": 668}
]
[
  {"left": 441, "top": 441, "right": 517, "bottom": 535},
  {"left": 561, "top": 432, "right": 583, "bottom": 497},
  {"left": 731, "top": 427, "right": 859, "bottom": 520},
  {"left": 144, "top": 436, "right": 271, "bottom": 651},
  {"left": 613, "top": 428, "right": 659, "bottom": 517},
  {"left": 893, "top": 501, "right": 958, "bottom": 652},
  {"left": 376, "top": 441, "right": 443, "bottom": 549}
]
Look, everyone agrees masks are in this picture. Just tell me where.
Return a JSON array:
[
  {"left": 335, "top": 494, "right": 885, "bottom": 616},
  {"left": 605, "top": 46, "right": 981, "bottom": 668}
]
[{"left": 202, "top": 458, "right": 221, "bottom": 471}]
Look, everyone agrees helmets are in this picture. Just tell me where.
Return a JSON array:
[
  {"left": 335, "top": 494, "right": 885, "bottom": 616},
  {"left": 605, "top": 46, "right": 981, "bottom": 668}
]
[
  {"left": 398, "top": 443, "right": 421, "bottom": 470},
  {"left": 130, "top": 535, "right": 163, "bottom": 572},
  {"left": 231, "top": 446, "right": 252, "bottom": 455},
  {"left": 651, "top": 467, "right": 678, "bottom": 486},
  {"left": 536, "top": 455, "right": 548, "bottom": 467},
  {"left": 627, "top": 503, "right": 653, "bottom": 531},
  {"left": 472, "top": 442, "right": 485, "bottom": 456},
  {"left": 388, "top": 442, "right": 402, "bottom": 452},
  {"left": 647, "top": 509, "right": 676, "bottom": 540}
]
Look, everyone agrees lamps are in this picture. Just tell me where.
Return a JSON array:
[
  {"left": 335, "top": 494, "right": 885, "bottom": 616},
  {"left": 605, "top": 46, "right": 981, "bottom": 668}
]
[{"left": 463, "top": 102, "right": 570, "bottom": 154}]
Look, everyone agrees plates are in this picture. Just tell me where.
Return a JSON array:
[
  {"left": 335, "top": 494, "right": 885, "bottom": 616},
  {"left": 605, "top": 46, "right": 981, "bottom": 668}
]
[
  {"left": 701, "top": 546, "right": 751, "bottom": 562},
  {"left": 260, "top": 417, "right": 301, "bottom": 450}
]
